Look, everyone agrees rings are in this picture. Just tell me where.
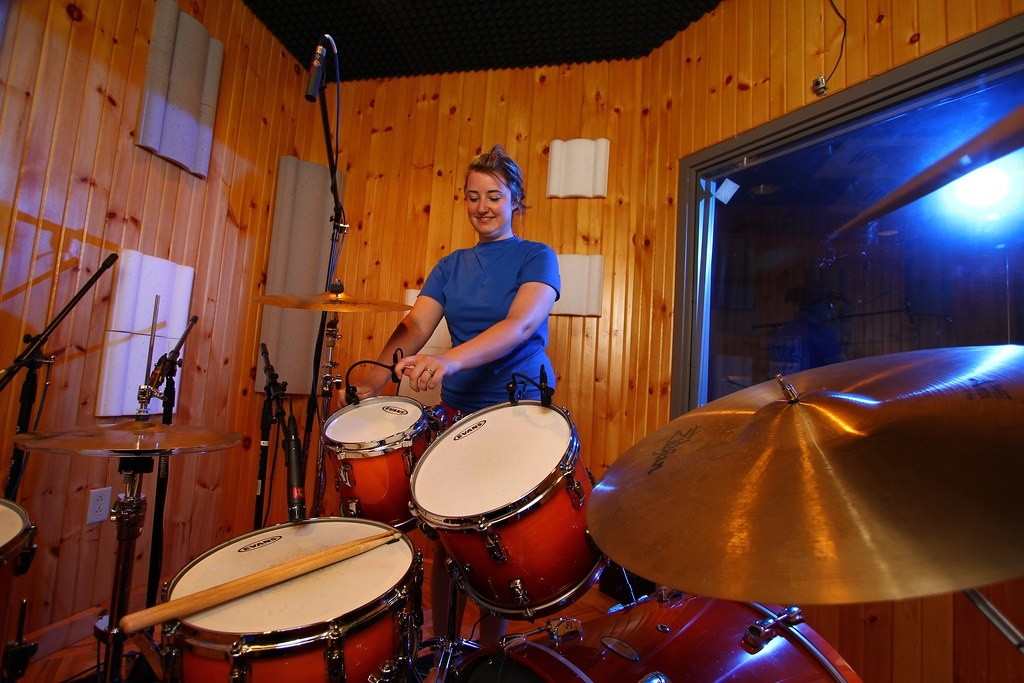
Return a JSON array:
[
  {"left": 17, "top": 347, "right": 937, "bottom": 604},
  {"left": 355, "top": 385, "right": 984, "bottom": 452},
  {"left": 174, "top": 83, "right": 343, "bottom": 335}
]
[{"left": 424, "top": 368, "right": 434, "bottom": 376}]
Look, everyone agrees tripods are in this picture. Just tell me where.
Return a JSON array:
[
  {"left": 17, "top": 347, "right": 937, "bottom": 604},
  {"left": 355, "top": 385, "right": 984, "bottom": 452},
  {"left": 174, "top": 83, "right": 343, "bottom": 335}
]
[{"left": 418, "top": 577, "right": 483, "bottom": 683}]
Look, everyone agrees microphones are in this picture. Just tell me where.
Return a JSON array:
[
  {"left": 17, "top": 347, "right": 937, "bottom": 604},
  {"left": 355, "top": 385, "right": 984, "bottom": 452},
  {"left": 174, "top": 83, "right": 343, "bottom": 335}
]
[
  {"left": 304, "top": 34, "right": 327, "bottom": 102},
  {"left": 391, "top": 353, "right": 402, "bottom": 383},
  {"left": 288, "top": 415, "right": 306, "bottom": 522},
  {"left": 541, "top": 364, "right": 552, "bottom": 407}
]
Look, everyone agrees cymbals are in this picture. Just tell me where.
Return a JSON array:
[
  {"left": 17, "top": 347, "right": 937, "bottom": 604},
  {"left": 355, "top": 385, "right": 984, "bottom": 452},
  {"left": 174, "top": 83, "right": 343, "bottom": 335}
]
[
  {"left": 10, "top": 420, "right": 243, "bottom": 459},
  {"left": 251, "top": 290, "right": 413, "bottom": 313},
  {"left": 584, "top": 343, "right": 1024, "bottom": 605}
]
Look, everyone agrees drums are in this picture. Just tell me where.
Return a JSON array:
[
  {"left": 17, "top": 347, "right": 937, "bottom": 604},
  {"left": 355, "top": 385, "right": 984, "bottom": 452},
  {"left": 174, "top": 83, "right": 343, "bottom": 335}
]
[
  {"left": 408, "top": 400, "right": 611, "bottom": 624},
  {"left": 319, "top": 395, "right": 430, "bottom": 534},
  {"left": 1, "top": 498, "right": 41, "bottom": 683},
  {"left": 438, "top": 589, "right": 864, "bottom": 683},
  {"left": 166, "top": 514, "right": 420, "bottom": 683}
]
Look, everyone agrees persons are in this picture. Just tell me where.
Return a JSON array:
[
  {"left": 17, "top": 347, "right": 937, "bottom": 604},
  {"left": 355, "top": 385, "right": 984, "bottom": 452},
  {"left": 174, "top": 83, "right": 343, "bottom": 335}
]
[
  {"left": 767, "top": 280, "right": 848, "bottom": 379},
  {"left": 336, "top": 142, "right": 562, "bottom": 683}
]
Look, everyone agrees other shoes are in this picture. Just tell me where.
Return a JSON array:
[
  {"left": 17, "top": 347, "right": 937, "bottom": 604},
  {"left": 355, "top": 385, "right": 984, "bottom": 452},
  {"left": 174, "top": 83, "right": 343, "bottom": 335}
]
[{"left": 422, "top": 666, "right": 439, "bottom": 683}]
